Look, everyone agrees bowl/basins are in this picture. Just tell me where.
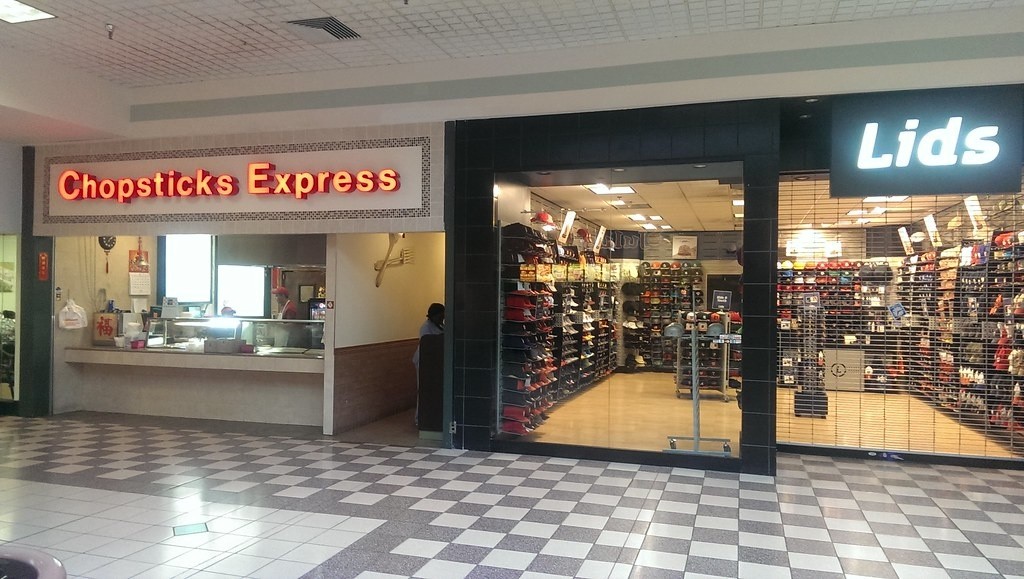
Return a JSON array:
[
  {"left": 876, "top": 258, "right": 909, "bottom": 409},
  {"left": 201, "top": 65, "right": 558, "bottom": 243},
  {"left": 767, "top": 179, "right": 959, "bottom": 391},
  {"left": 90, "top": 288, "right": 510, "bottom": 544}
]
[{"left": 114, "top": 334, "right": 125, "bottom": 347}]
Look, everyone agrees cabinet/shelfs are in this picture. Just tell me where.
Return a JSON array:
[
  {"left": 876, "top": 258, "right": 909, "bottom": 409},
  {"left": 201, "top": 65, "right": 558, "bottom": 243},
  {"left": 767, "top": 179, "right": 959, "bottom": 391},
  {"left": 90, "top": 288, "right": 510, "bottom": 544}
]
[
  {"left": 777, "top": 229, "right": 1024, "bottom": 445},
  {"left": 497, "top": 219, "right": 742, "bottom": 438}
]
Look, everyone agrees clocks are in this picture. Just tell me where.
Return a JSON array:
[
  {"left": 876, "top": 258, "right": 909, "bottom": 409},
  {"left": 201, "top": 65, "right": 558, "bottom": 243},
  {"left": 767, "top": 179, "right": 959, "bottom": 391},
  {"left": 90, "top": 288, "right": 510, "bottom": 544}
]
[{"left": 98, "top": 237, "right": 116, "bottom": 250}]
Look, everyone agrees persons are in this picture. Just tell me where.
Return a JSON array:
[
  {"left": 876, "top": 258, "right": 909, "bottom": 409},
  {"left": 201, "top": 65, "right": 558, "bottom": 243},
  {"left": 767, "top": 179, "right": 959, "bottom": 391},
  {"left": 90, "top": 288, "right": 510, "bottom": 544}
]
[
  {"left": 411, "top": 303, "right": 446, "bottom": 425},
  {"left": 273, "top": 288, "right": 298, "bottom": 347},
  {"left": 216, "top": 307, "right": 236, "bottom": 338}
]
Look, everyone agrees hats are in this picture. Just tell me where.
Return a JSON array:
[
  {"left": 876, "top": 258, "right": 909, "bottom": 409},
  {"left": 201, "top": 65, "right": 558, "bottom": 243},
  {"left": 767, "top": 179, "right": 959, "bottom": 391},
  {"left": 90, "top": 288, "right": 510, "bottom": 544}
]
[
  {"left": 664, "top": 310, "right": 743, "bottom": 388},
  {"left": 221, "top": 308, "right": 236, "bottom": 315},
  {"left": 530, "top": 211, "right": 554, "bottom": 226},
  {"left": 501, "top": 223, "right": 618, "bottom": 434},
  {"left": 621, "top": 260, "right": 704, "bottom": 367},
  {"left": 775, "top": 232, "right": 1024, "bottom": 438},
  {"left": 271, "top": 287, "right": 289, "bottom": 294},
  {"left": 428, "top": 303, "right": 445, "bottom": 313}
]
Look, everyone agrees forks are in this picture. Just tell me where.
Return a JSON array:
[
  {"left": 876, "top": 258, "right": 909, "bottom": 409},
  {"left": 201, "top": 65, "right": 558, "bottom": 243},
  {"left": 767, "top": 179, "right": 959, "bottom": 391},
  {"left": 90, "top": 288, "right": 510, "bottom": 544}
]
[{"left": 374, "top": 248, "right": 416, "bottom": 269}]
